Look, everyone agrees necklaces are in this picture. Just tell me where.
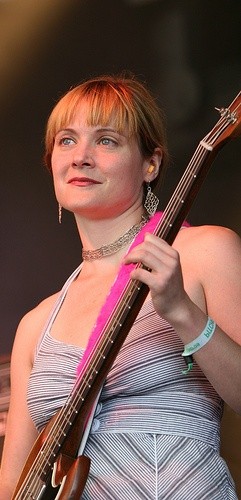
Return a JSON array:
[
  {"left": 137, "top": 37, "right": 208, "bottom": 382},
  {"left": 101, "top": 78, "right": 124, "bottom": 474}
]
[{"left": 82, "top": 216, "right": 148, "bottom": 262}]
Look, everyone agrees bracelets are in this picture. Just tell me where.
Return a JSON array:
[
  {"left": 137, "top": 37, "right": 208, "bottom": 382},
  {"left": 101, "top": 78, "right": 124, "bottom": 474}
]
[{"left": 181, "top": 316, "right": 218, "bottom": 376}]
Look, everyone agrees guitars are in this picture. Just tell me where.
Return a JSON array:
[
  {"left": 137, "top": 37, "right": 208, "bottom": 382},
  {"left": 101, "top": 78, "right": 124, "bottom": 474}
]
[{"left": 12, "top": 89, "right": 241, "bottom": 500}]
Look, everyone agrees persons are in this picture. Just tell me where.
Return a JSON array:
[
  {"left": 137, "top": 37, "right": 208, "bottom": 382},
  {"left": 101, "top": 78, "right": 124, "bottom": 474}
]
[{"left": 0, "top": 78, "right": 241, "bottom": 499}]
